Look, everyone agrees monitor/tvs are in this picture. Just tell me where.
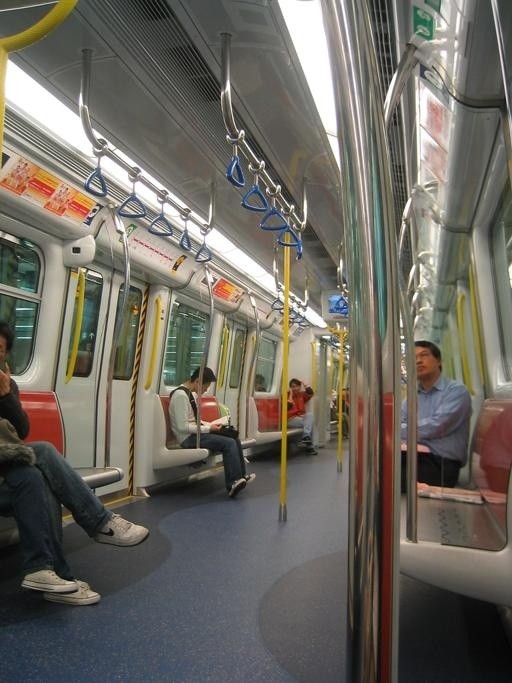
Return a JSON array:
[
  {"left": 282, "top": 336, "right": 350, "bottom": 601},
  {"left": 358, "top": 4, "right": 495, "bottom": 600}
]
[{"left": 320, "top": 289, "right": 350, "bottom": 322}]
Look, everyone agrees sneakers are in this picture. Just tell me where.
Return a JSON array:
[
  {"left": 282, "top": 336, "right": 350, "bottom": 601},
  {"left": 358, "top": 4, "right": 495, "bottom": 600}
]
[
  {"left": 41, "top": 579, "right": 101, "bottom": 606},
  {"left": 228, "top": 478, "right": 247, "bottom": 498},
  {"left": 21, "top": 570, "right": 79, "bottom": 594},
  {"left": 94, "top": 513, "right": 150, "bottom": 547},
  {"left": 244, "top": 472, "right": 256, "bottom": 485},
  {"left": 301, "top": 436, "right": 318, "bottom": 455}
]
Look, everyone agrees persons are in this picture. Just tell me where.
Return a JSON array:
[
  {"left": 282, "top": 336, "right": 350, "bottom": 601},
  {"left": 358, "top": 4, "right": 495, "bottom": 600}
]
[
  {"left": 398, "top": 340, "right": 470, "bottom": 488},
  {"left": 286, "top": 378, "right": 317, "bottom": 454},
  {"left": 254, "top": 374, "right": 268, "bottom": 393},
  {"left": 330, "top": 387, "right": 354, "bottom": 440},
  {"left": 0, "top": 320, "right": 149, "bottom": 607},
  {"left": 167, "top": 366, "right": 255, "bottom": 496}
]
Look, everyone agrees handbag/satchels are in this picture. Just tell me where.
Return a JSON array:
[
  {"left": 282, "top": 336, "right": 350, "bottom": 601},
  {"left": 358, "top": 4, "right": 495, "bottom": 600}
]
[{"left": 212, "top": 425, "right": 239, "bottom": 439}]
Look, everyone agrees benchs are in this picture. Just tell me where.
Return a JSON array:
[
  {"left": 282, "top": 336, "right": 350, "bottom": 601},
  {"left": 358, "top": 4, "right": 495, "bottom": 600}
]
[
  {"left": 149, "top": 388, "right": 305, "bottom": 470},
  {"left": 18, "top": 390, "right": 123, "bottom": 492},
  {"left": 400, "top": 398, "right": 512, "bottom": 610}
]
